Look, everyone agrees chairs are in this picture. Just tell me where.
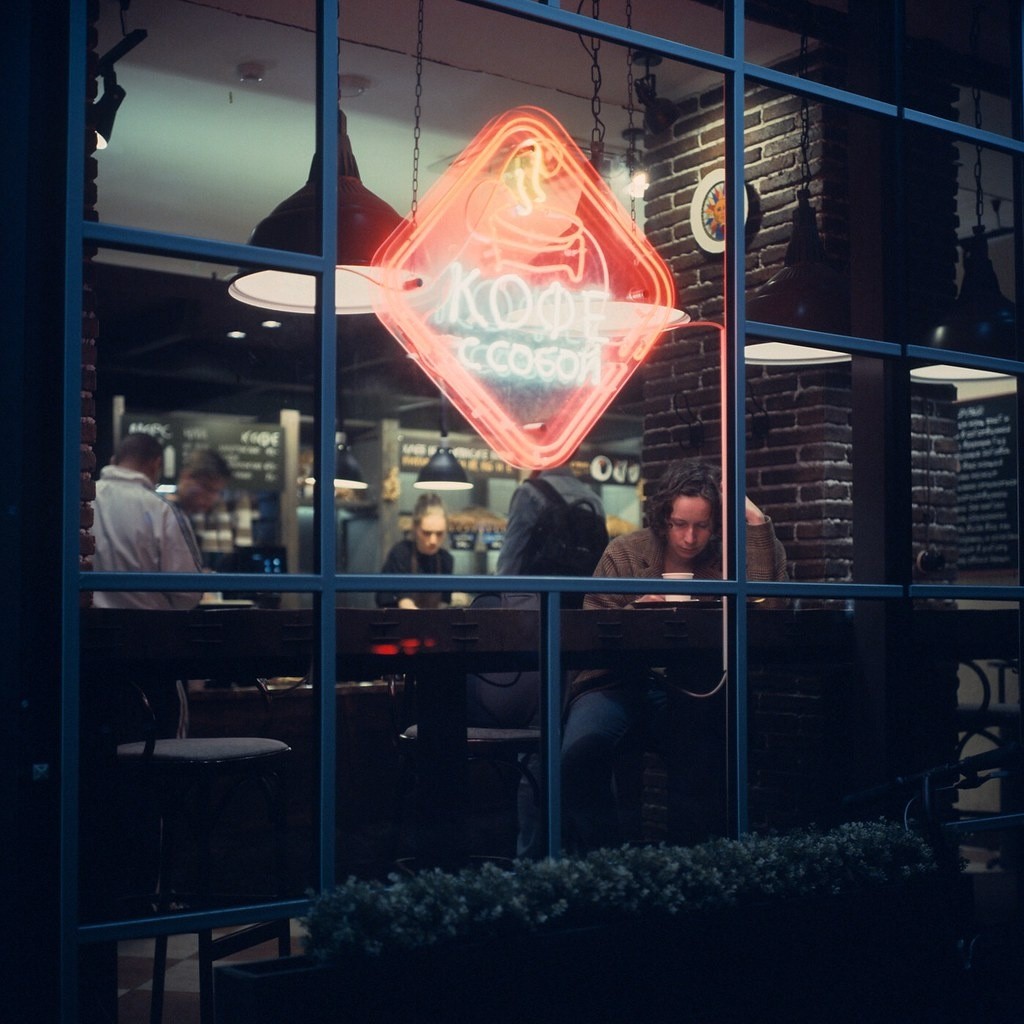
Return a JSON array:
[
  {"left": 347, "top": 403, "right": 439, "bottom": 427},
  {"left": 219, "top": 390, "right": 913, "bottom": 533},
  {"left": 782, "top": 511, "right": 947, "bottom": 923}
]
[{"left": 110, "top": 593, "right": 1022, "bottom": 1021}]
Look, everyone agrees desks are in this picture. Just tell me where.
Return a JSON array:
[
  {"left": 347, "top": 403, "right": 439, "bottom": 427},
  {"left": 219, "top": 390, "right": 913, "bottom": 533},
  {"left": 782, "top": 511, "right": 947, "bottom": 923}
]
[{"left": 84, "top": 606, "right": 1024, "bottom": 1023}]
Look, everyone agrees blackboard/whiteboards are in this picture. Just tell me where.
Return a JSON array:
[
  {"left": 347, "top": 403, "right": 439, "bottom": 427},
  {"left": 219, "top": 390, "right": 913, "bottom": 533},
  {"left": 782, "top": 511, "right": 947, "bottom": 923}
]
[{"left": 116, "top": 406, "right": 286, "bottom": 495}]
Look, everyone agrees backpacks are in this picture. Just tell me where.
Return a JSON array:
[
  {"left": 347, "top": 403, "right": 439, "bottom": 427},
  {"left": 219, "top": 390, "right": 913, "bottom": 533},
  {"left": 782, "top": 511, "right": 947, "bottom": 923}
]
[{"left": 517, "top": 478, "right": 613, "bottom": 613}]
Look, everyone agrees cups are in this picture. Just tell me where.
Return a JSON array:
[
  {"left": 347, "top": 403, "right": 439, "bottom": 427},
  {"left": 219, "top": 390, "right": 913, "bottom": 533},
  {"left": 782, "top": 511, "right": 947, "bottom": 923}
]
[
  {"left": 661, "top": 572, "right": 693, "bottom": 601},
  {"left": 194, "top": 497, "right": 254, "bottom": 555}
]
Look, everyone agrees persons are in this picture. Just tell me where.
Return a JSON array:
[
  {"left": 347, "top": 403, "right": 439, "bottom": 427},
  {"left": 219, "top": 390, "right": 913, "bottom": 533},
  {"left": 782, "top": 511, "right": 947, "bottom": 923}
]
[
  {"left": 375, "top": 492, "right": 454, "bottom": 608},
  {"left": 496, "top": 461, "right": 609, "bottom": 610},
  {"left": 91, "top": 431, "right": 207, "bottom": 611},
  {"left": 160, "top": 446, "right": 231, "bottom": 574},
  {"left": 562, "top": 462, "right": 792, "bottom": 857}
]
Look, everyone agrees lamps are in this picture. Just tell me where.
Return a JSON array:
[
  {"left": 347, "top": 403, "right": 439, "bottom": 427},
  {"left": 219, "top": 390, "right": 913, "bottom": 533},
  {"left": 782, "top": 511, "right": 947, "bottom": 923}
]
[
  {"left": 621, "top": 127, "right": 648, "bottom": 198},
  {"left": 227, "top": 1, "right": 432, "bottom": 317},
  {"left": 629, "top": 50, "right": 682, "bottom": 134},
  {"left": 906, "top": 0, "right": 1024, "bottom": 385},
  {"left": 742, "top": 0, "right": 856, "bottom": 368},
  {"left": 96, "top": 27, "right": 149, "bottom": 151},
  {"left": 414, "top": 391, "right": 474, "bottom": 491},
  {"left": 304, "top": 317, "right": 369, "bottom": 491}
]
